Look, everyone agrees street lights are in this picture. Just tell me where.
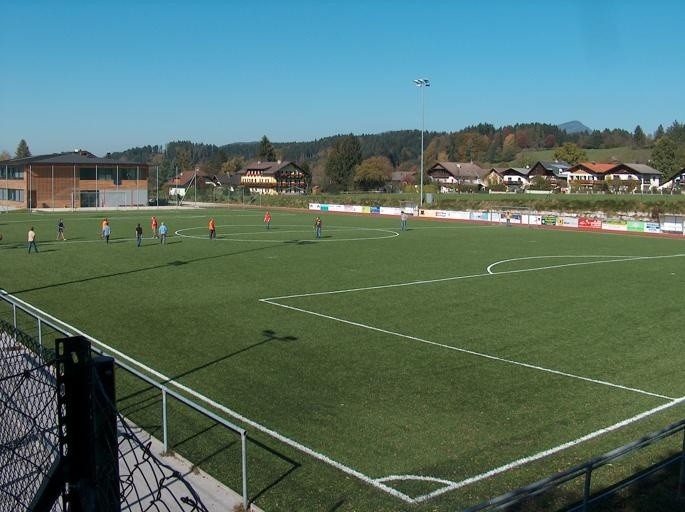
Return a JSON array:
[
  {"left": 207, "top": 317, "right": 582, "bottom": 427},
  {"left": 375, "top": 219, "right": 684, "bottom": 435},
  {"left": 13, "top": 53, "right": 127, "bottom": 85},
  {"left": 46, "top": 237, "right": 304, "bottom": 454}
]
[{"left": 413, "top": 78, "right": 432, "bottom": 206}]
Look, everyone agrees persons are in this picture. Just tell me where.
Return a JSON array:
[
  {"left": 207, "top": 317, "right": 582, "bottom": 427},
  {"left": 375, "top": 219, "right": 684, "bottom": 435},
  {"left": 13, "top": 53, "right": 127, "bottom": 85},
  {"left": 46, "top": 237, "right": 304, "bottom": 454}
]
[
  {"left": 26, "top": 226, "right": 39, "bottom": 254},
  {"left": 55, "top": 217, "right": 68, "bottom": 242},
  {"left": 102, "top": 221, "right": 112, "bottom": 245},
  {"left": 505, "top": 211, "right": 513, "bottom": 227},
  {"left": 263, "top": 210, "right": 271, "bottom": 231},
  {"left": 400, "top": 210, "right": 409, "bottom": 232},
  {"left": 208, "top": 217, "right": 217, "bottom": 241},
  {"left": 311, "top": 216, "right": 323, "bottom": 241},
  {"left": 159, "top": 221, "right": 168, "bottom": 246},
  {"left": 150, "top": 216, "right": 158, "bottom": 239},
  {"left": 135, "top": 222, "right": 143, "bottom": 247},
  {"left": 100, "top": 217, "right": 108, "bottom": 239}
]
